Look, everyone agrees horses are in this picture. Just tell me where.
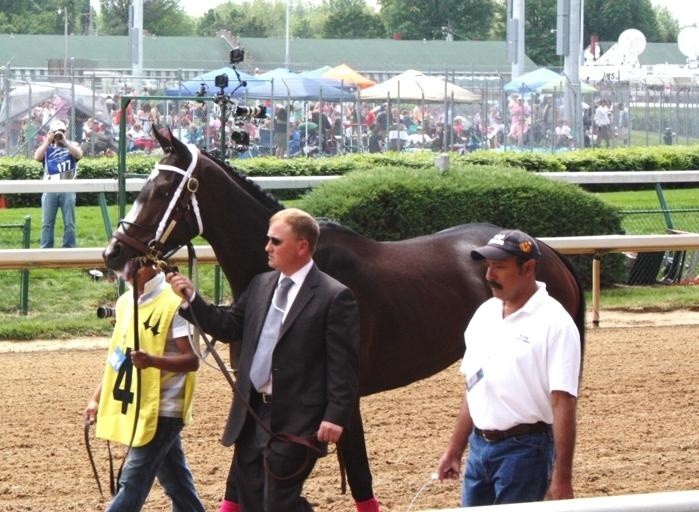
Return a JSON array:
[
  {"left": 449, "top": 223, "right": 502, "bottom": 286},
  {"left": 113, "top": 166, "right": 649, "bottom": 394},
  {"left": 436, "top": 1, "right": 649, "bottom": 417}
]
[{"left": 101, "top": 122, "right": 586, "bottom": 512}]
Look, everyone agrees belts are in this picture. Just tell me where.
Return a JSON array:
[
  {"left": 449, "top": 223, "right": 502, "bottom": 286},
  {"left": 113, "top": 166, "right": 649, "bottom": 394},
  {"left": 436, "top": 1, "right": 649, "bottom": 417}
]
[
  {"left": 474, "top": 424, "right": 546, "bottom": 442},
  {"left": 249, "top": 393, "right": 271, "bottom": 404}
]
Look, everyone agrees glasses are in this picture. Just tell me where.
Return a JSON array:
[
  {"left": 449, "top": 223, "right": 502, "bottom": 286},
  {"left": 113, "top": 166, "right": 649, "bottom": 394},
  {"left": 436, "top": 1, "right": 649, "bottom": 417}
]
[{"left": 263, "top": 235, "right": 301, "bottom": 244}]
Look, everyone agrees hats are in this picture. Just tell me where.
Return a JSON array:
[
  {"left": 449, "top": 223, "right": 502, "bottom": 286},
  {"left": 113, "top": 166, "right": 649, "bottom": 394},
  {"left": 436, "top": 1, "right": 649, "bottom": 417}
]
[
  {"left": 471, "top": 230, "right": 542, "bottom": 261},
  {"left": 50, "top": 120, "right": 65, "bottom": 131}
]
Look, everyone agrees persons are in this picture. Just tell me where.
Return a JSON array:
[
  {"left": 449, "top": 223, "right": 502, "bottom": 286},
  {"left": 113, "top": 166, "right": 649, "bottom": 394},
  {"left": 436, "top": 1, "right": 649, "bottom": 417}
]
[
  {"left": 36, "top": 119, "right": 82, "bottom": 248},
  {"left": 87, "top": 256, "right": 204, "bottom": 512},
  {"left": 437, "top": 230, "right": 580, "bottom": 507},
  {"left": 0, "top": 83, "right": 629, "bottom": 157},
  {"left": 166, "top": 208, "right": 359, "bottom": 512}
]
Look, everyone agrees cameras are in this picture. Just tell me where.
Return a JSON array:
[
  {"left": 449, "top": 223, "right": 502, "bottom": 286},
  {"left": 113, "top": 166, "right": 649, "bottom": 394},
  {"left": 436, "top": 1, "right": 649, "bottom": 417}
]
[
  {"left": 52, "top": 129, "right": 64, "bottom": 140},
  {"left": 236, "top": 105, "right": 266, "bottom": 117}
]
